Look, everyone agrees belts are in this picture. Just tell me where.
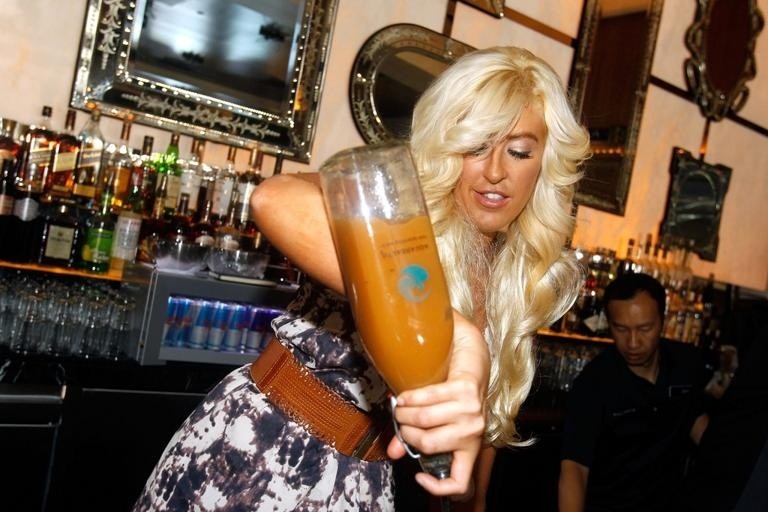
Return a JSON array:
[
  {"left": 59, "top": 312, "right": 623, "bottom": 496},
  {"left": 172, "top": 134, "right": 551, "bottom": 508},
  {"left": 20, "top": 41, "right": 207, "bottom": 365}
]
[{"left": 249, "top": 338, "right": 391, "bottom": 464}]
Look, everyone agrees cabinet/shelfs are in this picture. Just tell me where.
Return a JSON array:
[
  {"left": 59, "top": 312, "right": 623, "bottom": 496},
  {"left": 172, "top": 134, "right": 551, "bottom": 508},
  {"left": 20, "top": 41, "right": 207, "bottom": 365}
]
[{"left": 126, "top": 257, "right": 309, "bottom": 371}]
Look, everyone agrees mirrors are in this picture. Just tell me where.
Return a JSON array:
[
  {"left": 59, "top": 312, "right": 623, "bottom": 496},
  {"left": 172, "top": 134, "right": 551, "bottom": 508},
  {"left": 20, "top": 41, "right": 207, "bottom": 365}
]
[
  {"left": 678, "top": 1, "right": 764, "bottom": 122},
  {"left": 67, "top": 0, "right": 340, "bottom": 166},
  {"left": 657, "top": 144, "right": 733, "bottom": 263},
  {"left": 347, "top": 19, "right": 484, "bottom": 148},
  {"left": 560, "top": 1, "right": 664, "bottom": 217}
]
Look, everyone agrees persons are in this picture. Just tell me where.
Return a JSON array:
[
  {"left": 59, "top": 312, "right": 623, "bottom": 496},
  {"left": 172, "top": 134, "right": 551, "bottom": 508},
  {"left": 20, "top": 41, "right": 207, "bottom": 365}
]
[
  {"left": 126, "top": 43, "right": 595, "bottom": 512},
  {"left": 555, "top": 269, "right": 725, "bottom": 511},
  {"left": 705, "top": 342, "right": 740, "bottom": 399}
]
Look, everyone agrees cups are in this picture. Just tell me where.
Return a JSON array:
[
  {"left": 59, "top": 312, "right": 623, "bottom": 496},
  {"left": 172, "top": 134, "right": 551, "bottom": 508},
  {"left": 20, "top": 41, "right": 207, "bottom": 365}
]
[
  {"left": 1, "top": 269, "right": 141, "bottom": 330},
  {"left": 2, "top": 315, "right": 123, "bottom": 361}
]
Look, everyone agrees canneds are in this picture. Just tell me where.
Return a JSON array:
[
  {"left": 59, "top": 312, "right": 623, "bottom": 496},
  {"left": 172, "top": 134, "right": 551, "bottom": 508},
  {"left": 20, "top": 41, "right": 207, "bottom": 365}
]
[{"left": 161, "top": 296, "right": 287, "bottom": 353}]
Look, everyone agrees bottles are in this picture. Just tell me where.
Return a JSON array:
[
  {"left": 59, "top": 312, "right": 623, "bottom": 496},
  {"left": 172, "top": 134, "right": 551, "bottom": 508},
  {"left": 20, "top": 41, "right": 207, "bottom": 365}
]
[
  {"left": 2, "top": 100, "right": 293, "bottom": 280},
  {"left": 552, "top": 228, "right": 728, "bottom": 351},
  {"left": 317, "top": 135, "right": 461, "bottom": 512}
]
